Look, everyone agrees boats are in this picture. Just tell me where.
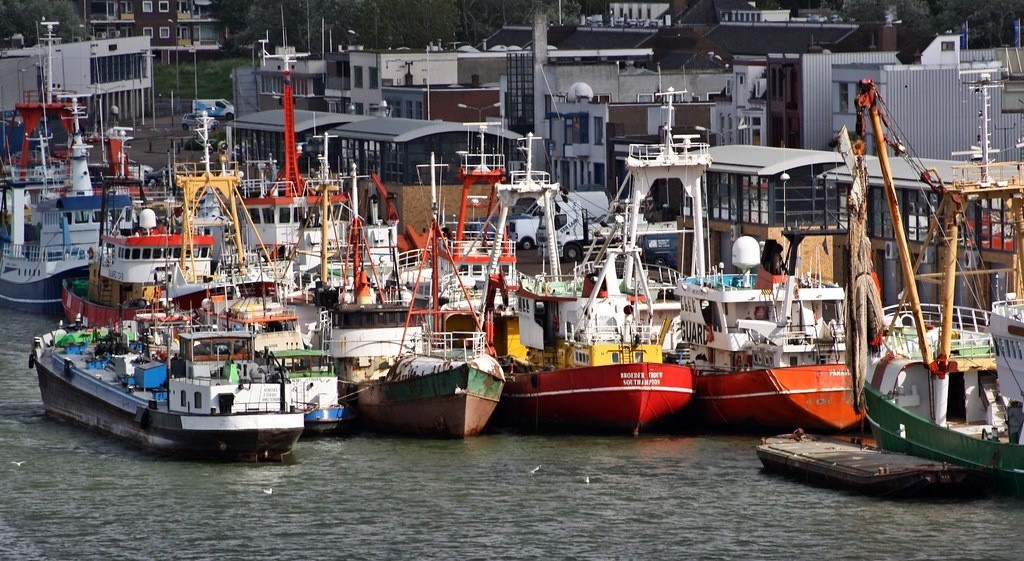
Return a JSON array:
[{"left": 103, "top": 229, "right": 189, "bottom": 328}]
[{"left": 0, "top": 17, "right": 1023, "bottom": 495}]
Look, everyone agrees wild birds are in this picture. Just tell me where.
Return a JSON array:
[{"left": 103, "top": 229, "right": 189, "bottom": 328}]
[
  {"left": 261, "top": 487, "right": 274, "bottom": 496},
  {"left": 10, "top": 459, "right": 29, "bottom": 468},
  {"left": 527, "top": 463, "right": 592, "bottom": 486}
]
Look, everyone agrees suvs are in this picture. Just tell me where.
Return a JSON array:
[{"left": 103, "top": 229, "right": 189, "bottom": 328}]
[{"left": 182, "top": 113, "right": 204, "bottom": 131}]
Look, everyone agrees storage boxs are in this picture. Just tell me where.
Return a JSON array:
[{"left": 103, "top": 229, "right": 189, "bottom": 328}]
[
  {"left": 178, "top": 39, "right": 191, "bottom": 46},
  {"left": 178, "top": 13, "right": 191, "bottom": 20},
  {"left": 120, "top": 13, "right": 134, "bottom": 20}
]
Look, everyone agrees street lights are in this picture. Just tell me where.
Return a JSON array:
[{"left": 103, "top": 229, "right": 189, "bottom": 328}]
[
  {"left": 54, "top": 48, "right": 66, "bottom": 91},
  {"left": 457, "top": 102, "right": 501, "bottom": 123},
  {"left": 87, "top": 83, "right": 127, "bottom": 130},
  {"left": 132, "top": 53, "right": 156, "bottom": 142},
  {"left": 140, "top": 47, "right": 161, "bottom": 132},
  {"left": 167, "top": 18, "right": 181, "bottom": 113},
  {"left": 16, "top": 55, "right": 34, "bottom": 102}
]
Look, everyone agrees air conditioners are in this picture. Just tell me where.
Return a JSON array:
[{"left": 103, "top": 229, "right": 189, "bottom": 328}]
[
  {"left": 110, "top": 31, "right": 120, "bottom": 38},
  {"left": 72, "top": 37, "right": 81, "bottom": 43},
  {"left": 85, "top": 36, "right": 95, "bottom": 41},
  {"left": 97, "top": 32, "right": 106, "bottom": 39}
]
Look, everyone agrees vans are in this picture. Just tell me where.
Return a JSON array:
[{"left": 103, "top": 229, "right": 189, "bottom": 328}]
[{"left": 192, "top": 97, "right": 234, "bottom": 121}]
